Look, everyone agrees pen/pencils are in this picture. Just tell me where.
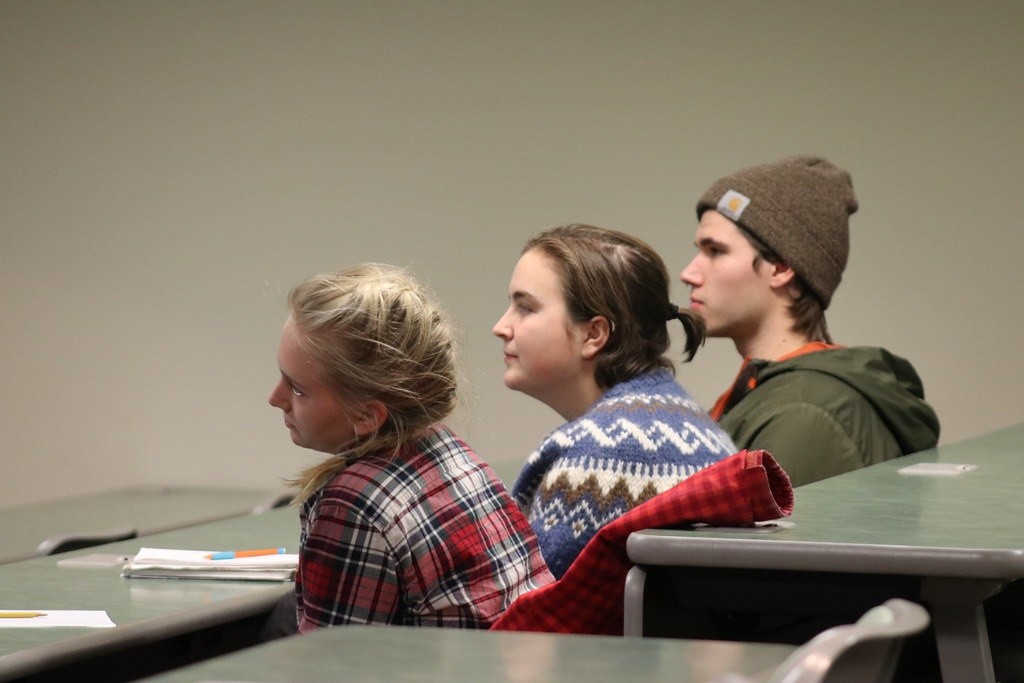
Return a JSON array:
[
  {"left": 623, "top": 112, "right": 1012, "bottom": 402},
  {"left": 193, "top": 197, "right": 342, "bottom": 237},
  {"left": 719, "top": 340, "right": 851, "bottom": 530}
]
[
  {"left": 0, "top": 612, "right": 47, "bottom": 618},
  {"left": 202, "top": 548, "right": 286, "bottom": 560}
]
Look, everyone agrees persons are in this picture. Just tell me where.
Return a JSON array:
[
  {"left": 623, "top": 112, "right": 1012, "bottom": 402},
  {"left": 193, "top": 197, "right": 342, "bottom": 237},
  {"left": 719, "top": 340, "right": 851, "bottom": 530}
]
[
  {"left": 672, "top": 151, "right": 947, "bottom": 512},
  {"left": 266, "top": 260, "right": 563, "bottom": 652},
  {"left": 487, "top": 224, "right": 760, "bottom": 597}
]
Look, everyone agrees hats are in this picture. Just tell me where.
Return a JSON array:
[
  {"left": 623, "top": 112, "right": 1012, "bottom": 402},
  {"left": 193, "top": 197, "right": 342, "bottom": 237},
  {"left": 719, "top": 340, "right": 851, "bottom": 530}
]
[{"left": 696, "top": 154, "right": 860, "bottom": 310}]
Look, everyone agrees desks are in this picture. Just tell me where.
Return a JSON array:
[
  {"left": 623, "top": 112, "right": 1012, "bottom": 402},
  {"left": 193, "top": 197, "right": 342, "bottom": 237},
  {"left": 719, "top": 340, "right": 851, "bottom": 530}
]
[{"left": 2, "top": 411, "right": 1024, "bottom": 682}]
